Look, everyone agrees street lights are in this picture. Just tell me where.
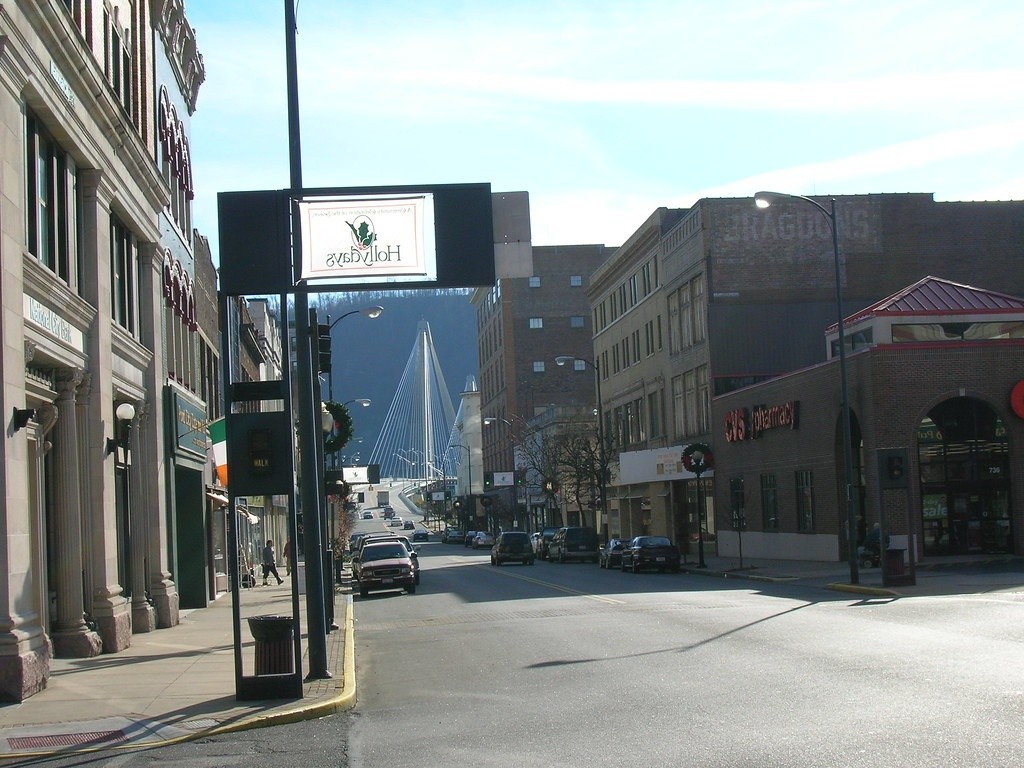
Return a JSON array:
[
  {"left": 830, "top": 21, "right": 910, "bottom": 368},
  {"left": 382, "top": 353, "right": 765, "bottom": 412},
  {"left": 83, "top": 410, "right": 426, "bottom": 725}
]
[
  {"left": 449, "top": 445, "right": 472, "bottom": 529},
  {"left": 754, "top": 192, "right": 858, "bottom": 583},
  {"left": 483, "top": 417, "right": 517, "bottom": 530},
  {"left": 555, "top": 355, "right": 608, "bottom": 545},
  {"left": 327, "top": 305, "right": 386, "bottom": 632},
  {"left": 691, "top": 450, "right": 708, "bottom": 568},
  {"left": 428, "top": 461, "right": 447, "bottom": 528}
]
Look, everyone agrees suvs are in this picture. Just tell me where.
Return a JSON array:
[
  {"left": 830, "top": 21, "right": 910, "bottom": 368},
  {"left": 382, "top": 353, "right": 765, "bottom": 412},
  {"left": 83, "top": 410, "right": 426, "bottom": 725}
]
[{"left": 547, "top": 527, "right": 600, "bottom": 561}]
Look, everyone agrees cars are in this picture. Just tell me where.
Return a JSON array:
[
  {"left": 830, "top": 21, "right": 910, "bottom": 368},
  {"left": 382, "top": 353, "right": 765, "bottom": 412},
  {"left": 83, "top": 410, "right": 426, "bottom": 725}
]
[
  {"left": 490, "top": 532, "right": 533, "bottom": 566},
  {"left": 621, "top": 535, "right": 681, "bottom": 573},
  {"left": 347, "top": 530, "right": 420, "bottom": 598},
  {"left": 598, "top": 538, "right": 632, "bottom": 569},
  {"left": 529, "top": 532, "right": 555, "bottom": 559},
  {"left": 471, "top": 532, "right": 495, "bottom": 549},
  {"left": 465, "top": 531, "right": 477, "bottom": 547}
]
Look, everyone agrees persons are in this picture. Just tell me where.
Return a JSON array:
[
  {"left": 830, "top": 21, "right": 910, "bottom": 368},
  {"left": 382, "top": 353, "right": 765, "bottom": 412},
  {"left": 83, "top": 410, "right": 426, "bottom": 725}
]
[
  {"left": 262, "top": 540, "right": 284, "bottom": 585},
  {"left": 871, "top": 522, "right": 890, "bottom": 548},
  {"left": 283, "top": 542, "right": 291, "bottom": 576}
]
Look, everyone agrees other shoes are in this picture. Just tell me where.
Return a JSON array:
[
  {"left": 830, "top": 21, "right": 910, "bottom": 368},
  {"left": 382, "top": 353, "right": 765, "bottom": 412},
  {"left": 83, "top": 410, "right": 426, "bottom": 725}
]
[{"left": 278, "top": 580, "right": 284, "bottom": 585}]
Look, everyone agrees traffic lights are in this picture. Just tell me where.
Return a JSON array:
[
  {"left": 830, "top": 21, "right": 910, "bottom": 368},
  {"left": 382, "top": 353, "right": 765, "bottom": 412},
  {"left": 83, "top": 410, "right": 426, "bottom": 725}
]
[
  {"left": 518, "top": 480, "right": 522, "bottom": 484},
  {"left": 485, "top": 481, "right": 490, "bottom": 485},
  {"left": 317, "top": 324, "right": 333, "bottom": 372}
]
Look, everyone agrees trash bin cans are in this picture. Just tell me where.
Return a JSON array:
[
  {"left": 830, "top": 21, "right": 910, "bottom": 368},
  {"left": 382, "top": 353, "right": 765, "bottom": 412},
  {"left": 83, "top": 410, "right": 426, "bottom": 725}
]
[
  {"left": 248, "top": 614, "right": 295, "bottom": 675},
  {"left": 883, "top": 547, "right": 905, "bottom": 587}
]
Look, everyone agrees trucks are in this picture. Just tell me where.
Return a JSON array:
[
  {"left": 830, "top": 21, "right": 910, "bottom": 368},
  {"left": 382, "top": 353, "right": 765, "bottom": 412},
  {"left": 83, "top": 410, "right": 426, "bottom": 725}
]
[
  {"left": 363, "top": 507, "right": 465, "bottom": 543},
  {"left": 376, "top": 491, "right": 389, "bottom": 507}
]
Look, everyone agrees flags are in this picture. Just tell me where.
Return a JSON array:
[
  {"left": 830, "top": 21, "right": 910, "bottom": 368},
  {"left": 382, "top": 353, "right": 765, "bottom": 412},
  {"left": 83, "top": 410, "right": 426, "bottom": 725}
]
[{"left": 208, "top": 417, "right": 228, "bottom": 487}]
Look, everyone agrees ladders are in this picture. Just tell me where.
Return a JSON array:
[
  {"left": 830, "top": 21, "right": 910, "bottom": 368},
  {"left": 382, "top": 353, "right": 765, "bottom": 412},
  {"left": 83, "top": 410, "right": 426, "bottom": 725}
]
[{"left": 238, "top": 538, "right": 253, "bottom": 591}]
